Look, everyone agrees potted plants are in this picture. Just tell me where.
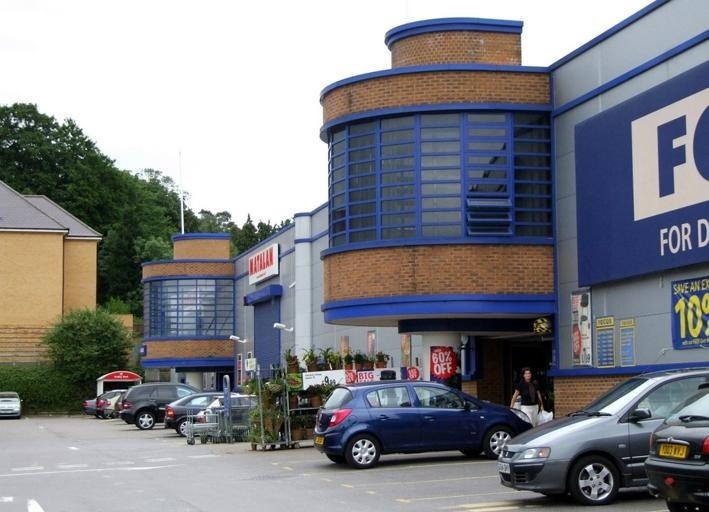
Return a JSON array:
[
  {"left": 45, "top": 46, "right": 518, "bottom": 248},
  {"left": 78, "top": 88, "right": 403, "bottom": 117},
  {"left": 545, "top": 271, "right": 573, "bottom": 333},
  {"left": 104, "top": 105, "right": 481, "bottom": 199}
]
[{"left": 242, "top": 343, "right": 390, "bottom": 450}]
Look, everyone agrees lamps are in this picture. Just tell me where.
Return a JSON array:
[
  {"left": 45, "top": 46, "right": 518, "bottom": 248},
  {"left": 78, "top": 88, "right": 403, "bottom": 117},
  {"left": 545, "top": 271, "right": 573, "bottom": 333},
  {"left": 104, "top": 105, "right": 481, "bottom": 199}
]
[
  {"left": 533, "top": 317, "right": 555, "bottom": 342},
  {"left": 229, "top": 335, "right": 246, "bottom": 344},
  {"left": 273, "top": 323, "right": 293, "bottom": 333}
]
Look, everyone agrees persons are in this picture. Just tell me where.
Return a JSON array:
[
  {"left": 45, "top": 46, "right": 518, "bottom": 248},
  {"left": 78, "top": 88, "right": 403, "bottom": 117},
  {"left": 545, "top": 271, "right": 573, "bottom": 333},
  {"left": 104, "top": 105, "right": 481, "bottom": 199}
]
[{"left": 509, "top": 367, "right": 544, "bottom": 426}]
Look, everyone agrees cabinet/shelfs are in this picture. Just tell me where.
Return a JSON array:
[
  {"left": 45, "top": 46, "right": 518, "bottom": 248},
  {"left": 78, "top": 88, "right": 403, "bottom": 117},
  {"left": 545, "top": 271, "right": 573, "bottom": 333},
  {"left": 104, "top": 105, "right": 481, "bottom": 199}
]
[{"left": 257, "top": 360, "right": 410, "bottom": 450}]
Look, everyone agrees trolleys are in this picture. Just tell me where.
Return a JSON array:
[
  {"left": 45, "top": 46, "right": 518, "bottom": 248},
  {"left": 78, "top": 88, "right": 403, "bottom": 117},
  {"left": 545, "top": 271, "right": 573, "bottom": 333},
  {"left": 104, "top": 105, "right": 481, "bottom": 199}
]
[{"left": 186, "top": 408, "right": 220, "bottom": 444}]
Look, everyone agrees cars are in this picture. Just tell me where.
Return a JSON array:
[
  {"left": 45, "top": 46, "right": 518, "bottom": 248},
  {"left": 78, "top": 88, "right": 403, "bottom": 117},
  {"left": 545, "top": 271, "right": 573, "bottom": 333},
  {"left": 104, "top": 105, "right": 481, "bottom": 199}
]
[
  {"left": 82, "top": 382, "right": 261, "bottom": 437},
  {"left": 314, "top": 380, "right": 533, "bottom": 469},
  {"left": 498, "top": 366, "right": 709, "bottom": 512},
  {"left": 0, "top": 391, "right": 24, "bottom": 419}
]
